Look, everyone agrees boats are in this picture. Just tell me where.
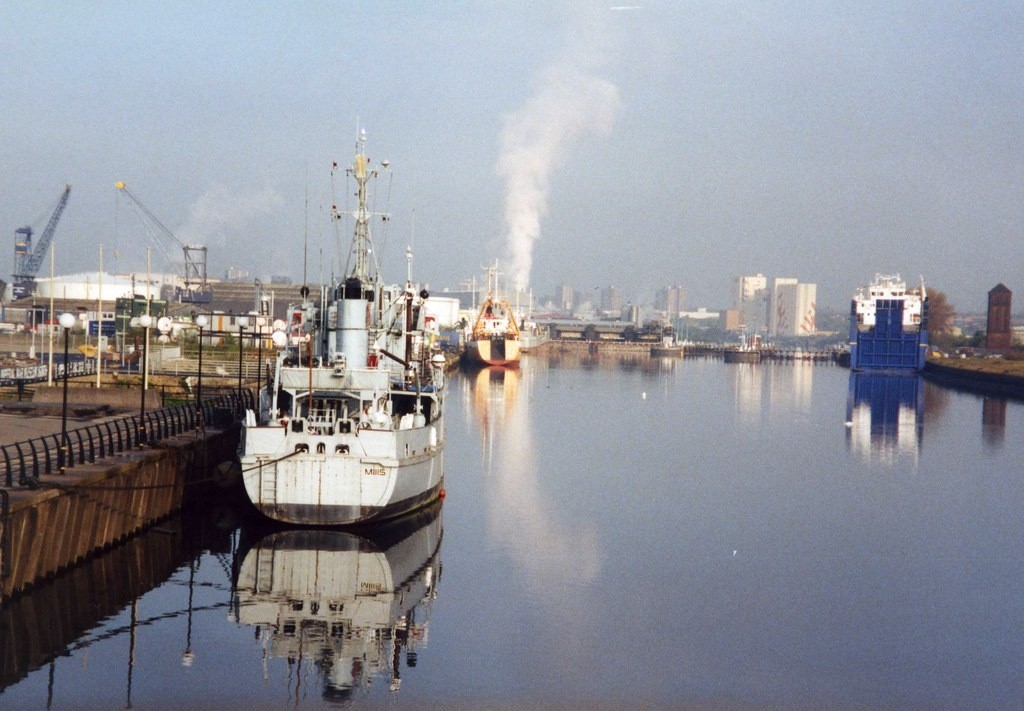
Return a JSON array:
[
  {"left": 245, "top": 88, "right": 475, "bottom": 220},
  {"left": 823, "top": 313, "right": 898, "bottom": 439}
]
[
  {"left": 232, "top": 116, "right": 449, "bottom": 527},
  {"left": 224, "top": 505, "right": 447, "bottom": 711},
  {"left": 460, "top": 260, "right": 524, "bottom": 367}
]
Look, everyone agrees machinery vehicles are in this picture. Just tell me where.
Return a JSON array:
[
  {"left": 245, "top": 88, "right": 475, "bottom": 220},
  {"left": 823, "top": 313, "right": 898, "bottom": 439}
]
[
  {"left": 115, "top": 179, "right": 215, "bottom": 306},
  {"left": 9, "top": 183, "right": 74, "bottom": 301}
]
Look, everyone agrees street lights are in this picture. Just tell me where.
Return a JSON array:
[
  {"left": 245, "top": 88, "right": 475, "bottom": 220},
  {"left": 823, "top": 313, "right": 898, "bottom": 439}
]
[
  {"left": 132, "top": 313, "right": 176, "bottom": 450},
  {"left": 183, "top": 554, "right": 200, "bottom": 670},
  {"left": 57, "top": 312, "right": 76, "bottom": 475},
  {"left": 195, "top": 315, "right": 208, "bottom": 437},
  {"left": 236, "top": 313, "right": 250, "bottom": 416},
  {"left": 254, "top": 315, "right": 266, "bottom": 418}
]
